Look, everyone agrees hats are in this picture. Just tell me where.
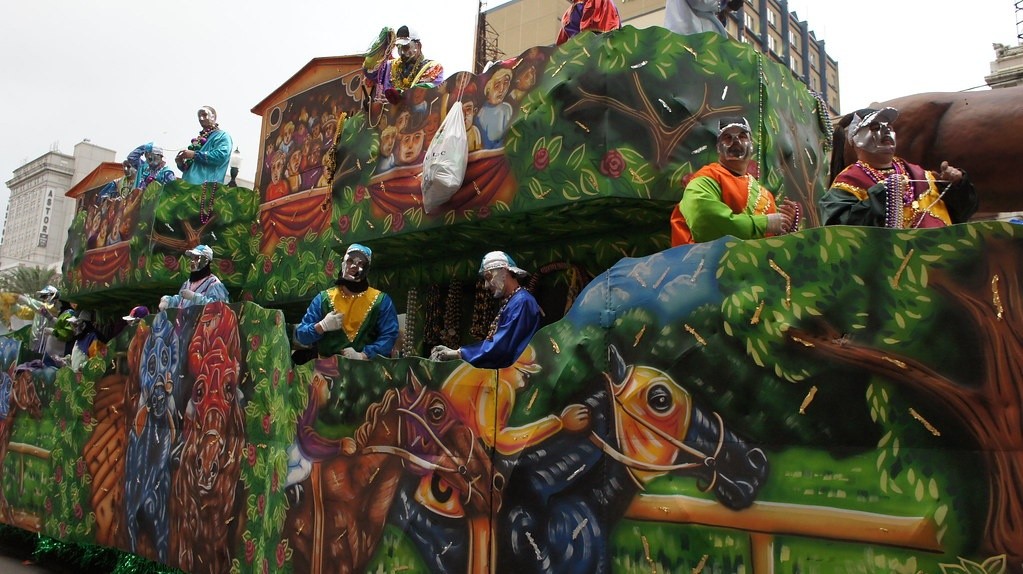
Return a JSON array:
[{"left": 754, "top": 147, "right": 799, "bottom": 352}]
[
  {"left": 393, "top": 26, "right": 420, "bottom": 46},
  {"left": 184, "top": 245, "right": 214, "bottom": 262},
  {"left": 122, "top": 306, "right": 149, "bottom": 321},
  {"left": 347, "top": 244, "right": 371, "bottom": 264},
  {"left": 37, "top": 286, "right": 59, "bottom": 297},
  {"left": 848, "top": 107, "right": 900, "bottom": 146},
  {"left": 66, "top": 311, "right": 91, "bottom": 323},
  {"left": 478, "top": 251, "right": 527, "bottom": 278},
  {"left": 717, "top": 116, "right": 751, "bottom": 141}
]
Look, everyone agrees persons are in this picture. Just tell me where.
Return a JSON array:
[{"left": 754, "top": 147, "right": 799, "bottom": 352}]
[
  {"left": 664, "top": 0, "right": 744, "bottom": 40},
  {"left": 430, "top": 250, "right": 542, "bottom": 369},
  {"left": 295, "top": 244, "right": 400, "bottom": 359},
  {"left": 122, "top": 306, "right": 151, "bottom": 324},
  {"left": 557, "top": 1, "right": 622, "bottom": 36},
  {"left": 822, "top": 105, "right": 974, "bottom": 230},
  {"left": 53, "top": 307, "right": 106, "bottom": 370},
  {"left": 158, "top": 244, "right": 229, "bottom": 309},
  {"left": 38, "top": 292, "right": 76, "bottom": 370},
  {"left": 671, "top": 116, "right": 803, "bottom": 246},
  {"left": 10, "top": 285, "right": 59, "bottom": 357}
]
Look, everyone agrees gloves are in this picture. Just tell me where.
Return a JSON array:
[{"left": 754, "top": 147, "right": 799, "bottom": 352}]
[
  {"left": 181, "top": 289, "right": 194, "bottom": 301},
  {"left": 44, "top": 326, "right": 55, "bottom": 335},
  {"left": 158, "top": 296, "right": 170, "bottom": 311},
  {"left": 431, "top": 345, "right": 461, "bottom": 361},
  {"left": 319, "top": 310, "right": 345, "bottom": 332},
  {"left": 344, "top": 347, "right": 367, "bottom": 360}
]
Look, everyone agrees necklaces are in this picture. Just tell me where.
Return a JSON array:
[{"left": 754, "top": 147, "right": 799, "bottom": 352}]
[
  {"left": 402, "top": 275, "right": 494, "bottom": 356},
  {"left": 776, "top": 200, "right": 798, "bottom": 236},
  {"left": 337, "top": 286, "right": 369, "bottom": 300},
  {"left": 856, "top": 154, "right": 952, "bottom": 230},
  {"left": 809, "top": 91, "right": 835, "bottom": 152},
  {"left": 484, "top": 284, "right": 528, "bottom": 342}
]
